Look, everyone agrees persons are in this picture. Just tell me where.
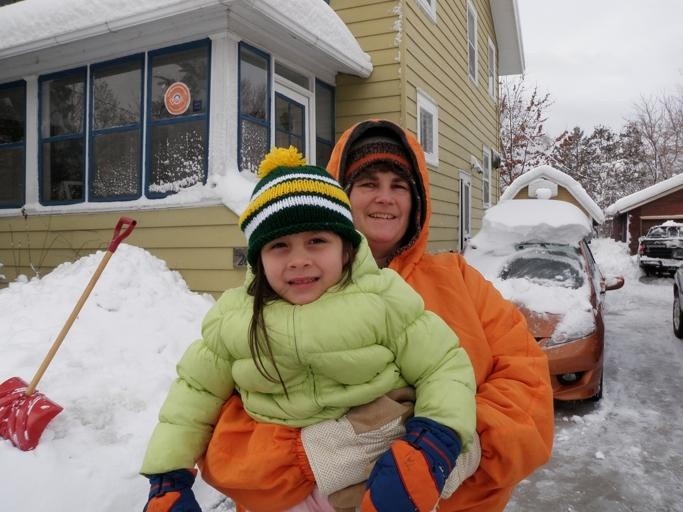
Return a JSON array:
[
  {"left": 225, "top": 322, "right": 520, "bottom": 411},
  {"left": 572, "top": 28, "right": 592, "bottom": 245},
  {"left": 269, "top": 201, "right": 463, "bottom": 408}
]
[
  {"left": 200, "top": 119, "right": 554, "bottom": 512},
  {"left": 137, "top": 146, "right": 477, "bottom": 512}
]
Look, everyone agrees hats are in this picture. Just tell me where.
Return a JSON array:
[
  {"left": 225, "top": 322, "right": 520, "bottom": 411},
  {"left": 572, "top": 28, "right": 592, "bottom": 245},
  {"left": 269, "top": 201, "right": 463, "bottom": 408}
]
[
  {"left": 238, "top": 147, "right": 361, "bottom": 269},
  {"left": 342, "top": 135, "right": 421, "bottom": 263}
]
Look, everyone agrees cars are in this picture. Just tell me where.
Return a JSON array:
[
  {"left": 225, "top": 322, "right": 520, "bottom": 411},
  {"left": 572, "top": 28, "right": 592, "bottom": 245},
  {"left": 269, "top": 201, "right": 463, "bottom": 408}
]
[
  {"left": 638, "top": 224, "right": 683, "bottom": 339},
  {"left": 462, "top": 225, "right": 624, "bottom": 402}
]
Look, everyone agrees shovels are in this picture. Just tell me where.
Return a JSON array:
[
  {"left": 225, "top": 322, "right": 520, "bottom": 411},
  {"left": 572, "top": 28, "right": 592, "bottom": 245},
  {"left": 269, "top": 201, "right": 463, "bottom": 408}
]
[{"left": 0, "top": 217, "right": 138, "bottom": 452}]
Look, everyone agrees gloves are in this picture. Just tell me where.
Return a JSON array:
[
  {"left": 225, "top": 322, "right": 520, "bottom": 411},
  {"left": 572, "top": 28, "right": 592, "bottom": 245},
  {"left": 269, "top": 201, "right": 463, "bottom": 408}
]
[
  {"left": 300, "top": 386, "right": 416, "bottom": 498},
  {"left": 360, "top": 417, "right": 463, "bottom": 512},
  {"left": 142, "top": 469, "right": 202, "bottom": 512},
  {"left": 328, "top": 429, "right": 482, "bottom": 511}
]
